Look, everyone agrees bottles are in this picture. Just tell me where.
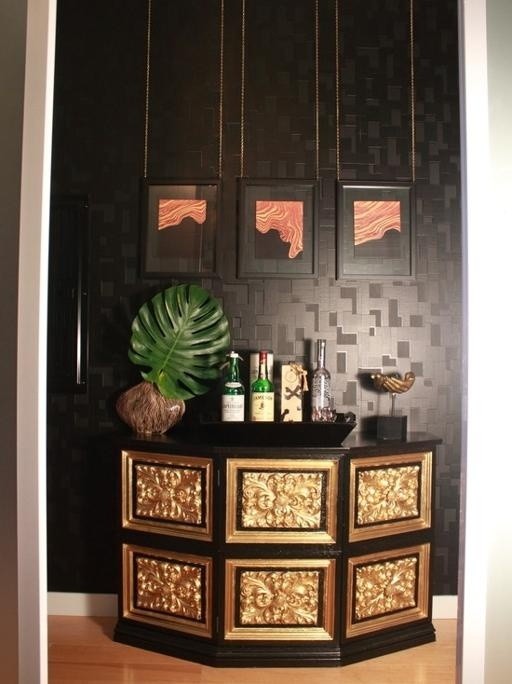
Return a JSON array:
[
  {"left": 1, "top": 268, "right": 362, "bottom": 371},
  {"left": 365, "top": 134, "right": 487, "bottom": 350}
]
[
  {"left": 220, "top": 351, "right": 245, "bottom": 422},
  {"left": 247, "top": 352, "right": 275, "bottom": 423},
  {"left": 310, "top": 339, "right": 334, "bottom": 422}
]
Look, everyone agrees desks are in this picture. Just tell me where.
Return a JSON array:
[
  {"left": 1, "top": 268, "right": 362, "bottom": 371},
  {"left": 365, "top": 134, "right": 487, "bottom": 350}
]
[{"left": 114, "top": 432, "right": 444, "bottom": 668}]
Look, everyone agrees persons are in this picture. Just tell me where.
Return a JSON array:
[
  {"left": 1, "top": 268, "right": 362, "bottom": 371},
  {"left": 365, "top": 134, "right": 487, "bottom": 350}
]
[{"left": 369, "top": 371, "right": 415, "bottom": 394}]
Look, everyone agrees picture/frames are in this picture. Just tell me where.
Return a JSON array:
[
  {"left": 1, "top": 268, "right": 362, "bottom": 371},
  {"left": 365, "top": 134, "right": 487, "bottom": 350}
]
[
  {"left": 236, "top": 173, "right": 319, "bottom": 279},
  {"left": 138, "top": 176, "right": 224, "bottom": 281},
  {"left": 334, "top": 181, "right": 417, "bottom": 282}
]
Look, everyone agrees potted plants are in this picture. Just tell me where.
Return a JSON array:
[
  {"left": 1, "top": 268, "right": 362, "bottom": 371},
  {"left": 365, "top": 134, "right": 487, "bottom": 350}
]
[{"left": 115, "top": 283, "right": 230, "bottom": 439}]
